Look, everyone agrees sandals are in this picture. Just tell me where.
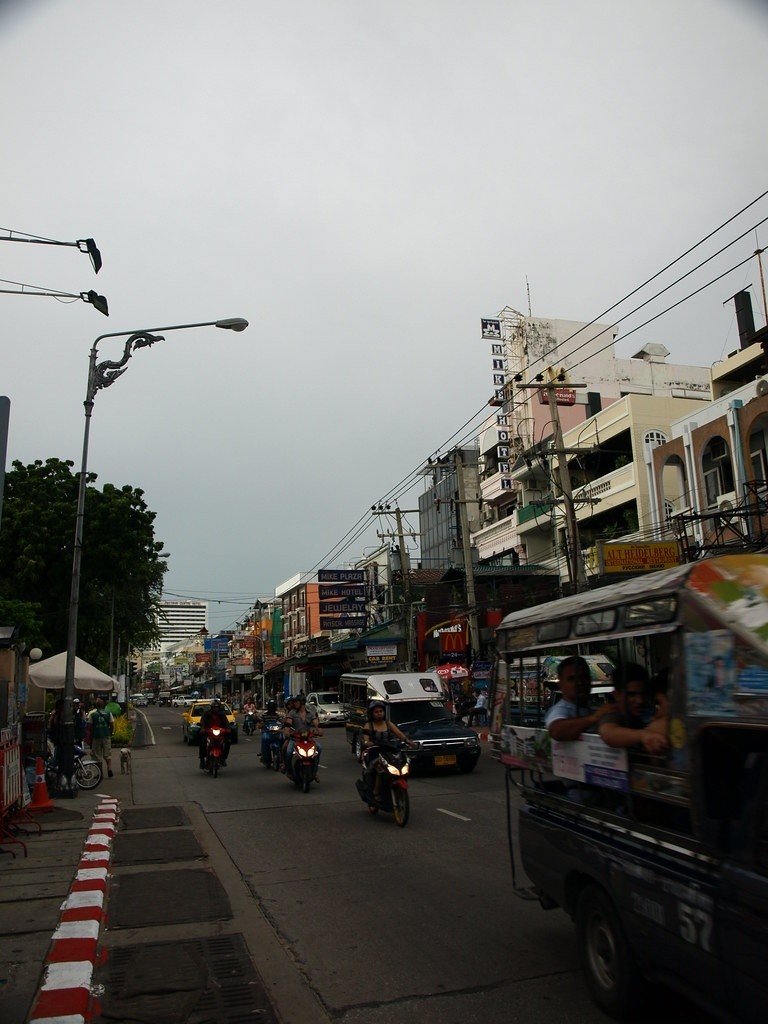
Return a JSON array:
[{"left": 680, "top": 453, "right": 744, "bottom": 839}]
[{"left": 372, "top": 791, "right": 381, "bottom": 802}]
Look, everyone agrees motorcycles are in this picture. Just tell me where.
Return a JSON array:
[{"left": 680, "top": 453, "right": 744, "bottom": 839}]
[
  {"left": 195, "top": 721, "right": 234, "bottom": 779},
  {"left": 486, "top": 553, "right": 768, "bottom": 1024},
  {"left": 241, "top": 708, "right": 259, "bottom": 736},
  {"left": 353, "top": 725, "right": 422, "bottom": 829},
  {"left": 257, "top": 715, "right": 285, "bottom": 772},
  {"left": 20, "top": 709, "right": 103, "bottom": 791},
  {"left": 280, "top": 721, "right": 322, "bottom": 793}
]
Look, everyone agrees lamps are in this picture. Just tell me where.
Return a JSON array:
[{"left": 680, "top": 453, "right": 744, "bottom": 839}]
[
  {"left": 0, "top": 228, "right": 102, "bottom": 275},
  {"left": 0, "top": 279, "right": 109, "bottom": 317}
]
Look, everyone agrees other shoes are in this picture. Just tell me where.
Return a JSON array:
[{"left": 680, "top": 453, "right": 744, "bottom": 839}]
[
  {"left": 314, "top": 775, "right": 320, "bottom": 783},
  {"left": 108, "top": 770, "right": 113, "bottom": 777},
  {"left": 94, "top": 775, "right": 103, "bottom": 779}
]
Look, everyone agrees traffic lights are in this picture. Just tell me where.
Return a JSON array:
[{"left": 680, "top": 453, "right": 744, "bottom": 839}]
[
  {"left": 257, "top": 661, "right": 263, "bottom": 674},
  {"left": 129, "top": 661, "right": 137, "bottom": 678}
]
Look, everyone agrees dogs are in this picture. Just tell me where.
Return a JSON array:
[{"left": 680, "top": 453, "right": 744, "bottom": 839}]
[{"left": 119, "top": 748, "right": 132, "bottom": 776}]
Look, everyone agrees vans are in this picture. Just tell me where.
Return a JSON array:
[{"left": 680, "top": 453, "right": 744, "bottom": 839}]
[{"left": 499, "top": 653, "right": 618, "bottom": 704}]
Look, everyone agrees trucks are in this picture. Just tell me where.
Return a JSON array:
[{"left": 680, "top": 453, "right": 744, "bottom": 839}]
[{"left": 337, "top": 671, "right": 482, "bottom": 779}]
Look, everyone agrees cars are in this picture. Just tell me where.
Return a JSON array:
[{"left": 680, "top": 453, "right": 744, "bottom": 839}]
[
  {"left": 305, "top": 691, "right": 345, "bottom": 728},
  {"left": 172, "top": 696, "right": 194, "bottom": 709},
  {"left": 129, "top": 691, "right": 172, "bottom": 709},
  {"left": 181, "top": 700, "right": 239, "bottom": 745}
]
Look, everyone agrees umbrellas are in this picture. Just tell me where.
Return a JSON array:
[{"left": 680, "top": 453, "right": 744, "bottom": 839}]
[
  {"left": 28, "top": 650, "right": 121, "bottom": 694},
  {"left": 433, "top": 663, "right": 470, "bottom": 698}
]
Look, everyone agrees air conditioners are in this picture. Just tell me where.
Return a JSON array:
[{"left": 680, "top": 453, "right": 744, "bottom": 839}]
[
  {"left": 482, "top": 510, "right": 494, "bottom": 521},
  {"left": 670, "top": 506, "right": 693, "bottom": 539},
  {"left": 754, "top": 373, "right": 768, "bottom": 397},
  {"left": 716, "top": 490, "right": 738, "bottom": 527},
  {"left": 477, "top": 455, "right": 490, "bottom": 475},
  {"left": 525, "top": 479, "right": 542, "bottom": 491}
]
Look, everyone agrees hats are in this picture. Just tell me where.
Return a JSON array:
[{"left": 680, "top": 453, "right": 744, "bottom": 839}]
[{"left": 368, "top": 701, "right": 385, "bottom": 711}]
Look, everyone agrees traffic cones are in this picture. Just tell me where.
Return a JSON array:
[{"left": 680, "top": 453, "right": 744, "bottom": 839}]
[{"left": 23, "top": 756, "right": 55, "bottom": 815}]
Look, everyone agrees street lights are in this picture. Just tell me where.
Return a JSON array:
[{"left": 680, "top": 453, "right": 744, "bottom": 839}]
[
  {"left": 107, "top": 550, "right": 171, "bottom": 703},
  {"left": 53, "top": 315, "right": 251, "bottom": 800},
  {"left": 126, "top": 628, "right": 172, "bottom": 710}
]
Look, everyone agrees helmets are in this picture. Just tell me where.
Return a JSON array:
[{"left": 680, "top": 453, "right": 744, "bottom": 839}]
[
  {"left": 211, "top": 700, "right": 221, "bottom": 713},
  {"left": 284, "top": 697, "right": 293, "bottom": 707},
  {"left": 294, "top": 694, "right": 306, "bottom": 704},
  {"left": 267, "top": 699, "right": 276, "bottom": 713}
]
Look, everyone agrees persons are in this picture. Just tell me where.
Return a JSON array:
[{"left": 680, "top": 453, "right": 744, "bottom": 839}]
[
  {"left": 217, "top": 689, "right": 261, "bottom": 731},
  {"left": 199, "top": 700, "right": 232, "bottom": 769},
  {"left": 545, "top": 656, "right": 681, "bottom": 751},
  {"left": 281, "top": 695, "right": 324, "bottom": 783},
  {"left": 363, "top": 702, "right": 417, "bottom": 802},
  {"left": 455, "top": 684, "right": 487, "bottom": 727},
  {"left": 50, "top": 698, "right": 114, "bottom": 777},
  {"left": 257, "top": 699, "right": 286, "bottom": 764}
]
